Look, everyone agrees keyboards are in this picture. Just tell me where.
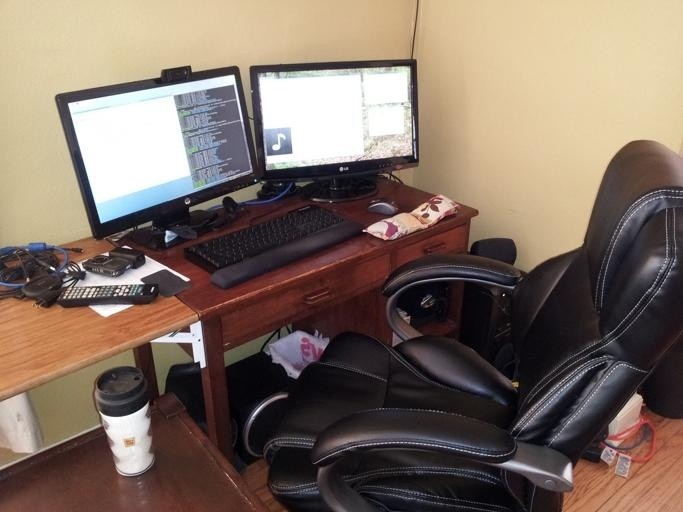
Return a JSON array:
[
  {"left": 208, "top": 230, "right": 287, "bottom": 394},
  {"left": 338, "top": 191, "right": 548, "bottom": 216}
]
[{"left": 183, "top": 203, "right": 367, "bottom": 289}]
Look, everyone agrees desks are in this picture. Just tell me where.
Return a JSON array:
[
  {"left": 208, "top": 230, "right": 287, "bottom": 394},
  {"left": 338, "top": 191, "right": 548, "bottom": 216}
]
[
  {"left": 0, "top": 233, "right": 201, "bottom": 402},
  {"left": 0, "top": 393, "right": 271, "bottom": 512},
  {"left": 107, "top": 171, "right": 479, "bottom": 458}
]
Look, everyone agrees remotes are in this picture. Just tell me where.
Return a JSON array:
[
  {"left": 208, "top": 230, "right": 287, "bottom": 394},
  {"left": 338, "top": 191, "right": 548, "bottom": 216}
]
[{"left": 56, "top": 282, "right": 159, "bottom": 308}]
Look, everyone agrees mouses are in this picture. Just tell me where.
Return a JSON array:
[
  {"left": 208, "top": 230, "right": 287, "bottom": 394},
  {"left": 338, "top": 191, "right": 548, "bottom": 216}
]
[{"left": 367, "top": 197, "right": 399, "bottom": 215}]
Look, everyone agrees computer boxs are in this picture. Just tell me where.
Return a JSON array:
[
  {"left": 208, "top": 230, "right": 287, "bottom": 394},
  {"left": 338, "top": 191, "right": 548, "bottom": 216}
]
[{"left": 403, "top": 276, "right": 452, "bottom": 327}]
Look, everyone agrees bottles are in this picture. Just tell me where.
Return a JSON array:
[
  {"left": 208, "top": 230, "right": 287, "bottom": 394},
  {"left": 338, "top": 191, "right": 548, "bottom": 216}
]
[{"left": 92, "top": 366, "right": 155, "bottom": 478}]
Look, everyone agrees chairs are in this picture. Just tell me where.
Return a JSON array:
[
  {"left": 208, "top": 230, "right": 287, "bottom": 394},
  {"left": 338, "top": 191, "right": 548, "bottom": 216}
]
[{"left": 243, "top": 141, "right": 683, "bottom": 512}]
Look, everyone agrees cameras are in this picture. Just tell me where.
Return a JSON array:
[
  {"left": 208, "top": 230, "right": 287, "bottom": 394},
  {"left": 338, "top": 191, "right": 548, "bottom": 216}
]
[{"left": 82, "top": 254, "right": 134, "bottom": 277}]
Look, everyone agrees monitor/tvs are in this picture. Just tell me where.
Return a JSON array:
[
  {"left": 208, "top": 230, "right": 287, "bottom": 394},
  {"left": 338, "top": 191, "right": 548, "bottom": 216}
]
[
  {"left": 249, "top": 58, "right": 420, "bottom": 203},
  {"left": 54, "top": 64, "right": 262, "bottom": 252}
]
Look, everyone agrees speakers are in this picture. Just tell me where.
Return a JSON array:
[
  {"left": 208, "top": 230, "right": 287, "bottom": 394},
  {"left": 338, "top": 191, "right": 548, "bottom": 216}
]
[{"left": 165, "top": 361, "right": 208, "bottom": 436}]
